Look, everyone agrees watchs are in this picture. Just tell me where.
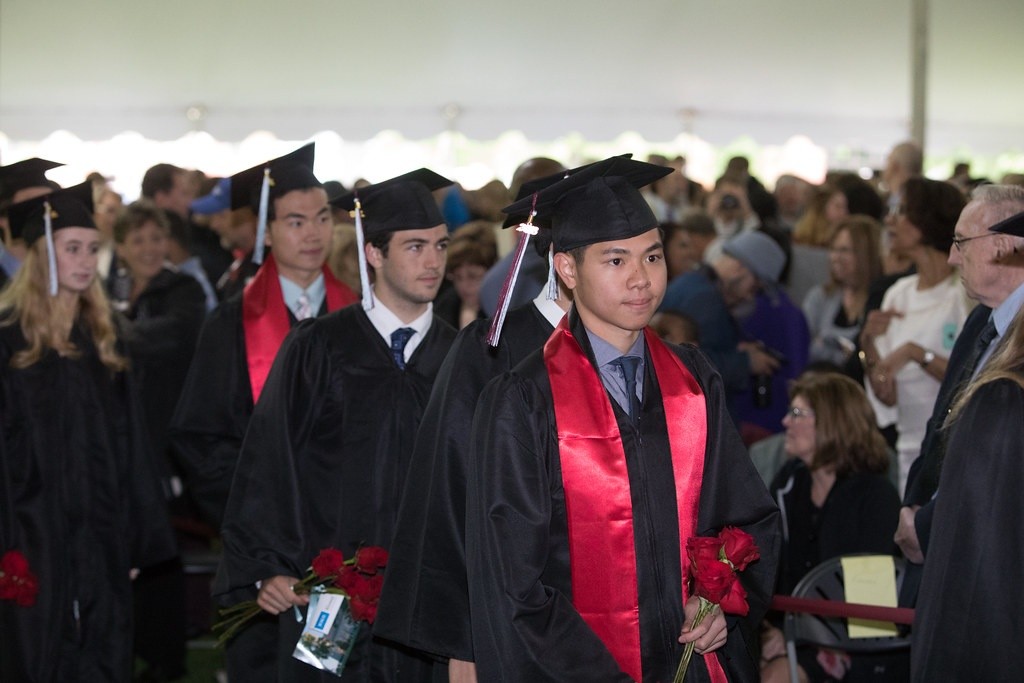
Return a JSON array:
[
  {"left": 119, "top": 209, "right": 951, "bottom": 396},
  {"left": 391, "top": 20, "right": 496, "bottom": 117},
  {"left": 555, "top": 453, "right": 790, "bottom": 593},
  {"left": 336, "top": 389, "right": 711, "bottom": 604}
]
[{"left": 921, "top": 350, "right": 934, "bottom": 368}]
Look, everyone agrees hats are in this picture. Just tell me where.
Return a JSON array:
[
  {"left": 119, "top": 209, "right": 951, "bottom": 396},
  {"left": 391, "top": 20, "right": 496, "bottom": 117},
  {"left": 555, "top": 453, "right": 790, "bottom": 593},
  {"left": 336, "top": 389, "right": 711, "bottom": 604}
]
[
  {"left": 726, "top": 230, "right": 785, "bottom": 281},
  {"left": 500, "top": 152, "right": 633, "bottom": 301},
  {"left": 486, "top": 156, "right": 677, "bottom": 347},
  {"left": 191, "top": 180, "right": 235, "bottom": 214},
  {"left": 4, "top": 179, "right": 96, "bottom": 298},
  {"left": 230, "top": 141, "right": 322, "bottom": 264},
  {"left": 1, "top": 157, "right": 66, "bottom": 209},
  {"left": 327, "top": 166, "right": 455, "bottom": 313},
  {"left": 988, "top": 212, "right": 1024, "bottom": 240}
]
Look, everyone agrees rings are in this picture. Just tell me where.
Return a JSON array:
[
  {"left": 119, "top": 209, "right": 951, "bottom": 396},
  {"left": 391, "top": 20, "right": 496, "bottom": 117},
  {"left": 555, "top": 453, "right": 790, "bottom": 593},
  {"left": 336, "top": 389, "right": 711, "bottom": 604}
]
[{"left": 877, "top": 375, "right": 886, "bottom": 382}]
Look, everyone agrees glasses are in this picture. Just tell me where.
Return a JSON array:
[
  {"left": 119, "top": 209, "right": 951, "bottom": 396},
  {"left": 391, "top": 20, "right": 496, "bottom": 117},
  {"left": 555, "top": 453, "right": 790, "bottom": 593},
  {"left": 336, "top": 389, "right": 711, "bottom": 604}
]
[
  {"left": 952, "top": 231, "right": 1003, "bottom": 252},
  {"left": 787, "top": 407, "right": 818, "bottom": 424}
]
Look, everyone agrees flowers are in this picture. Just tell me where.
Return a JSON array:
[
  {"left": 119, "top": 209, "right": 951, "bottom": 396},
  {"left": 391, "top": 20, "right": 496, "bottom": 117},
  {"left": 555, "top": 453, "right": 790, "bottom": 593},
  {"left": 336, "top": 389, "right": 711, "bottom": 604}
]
[
  {"left": 0, "top": 550, "right": 40, "bottom": 608},
  {"left": 209, "top": 546, "right": 388, "bottom": 652},
  {"left": 673, "top": 526, "right": 758, "bottom": 683}
]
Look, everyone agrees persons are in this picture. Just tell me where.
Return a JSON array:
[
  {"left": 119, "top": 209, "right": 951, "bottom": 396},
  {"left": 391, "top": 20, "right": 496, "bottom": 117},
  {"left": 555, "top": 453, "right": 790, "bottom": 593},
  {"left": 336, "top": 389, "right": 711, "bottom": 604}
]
[
  {"left": 215, "top": 169, "right": 457, "bottom": 683},
  {"left": 0, "top": 143, "right": 1024, "bottom": 683},
  {"left": 465, "top": 157, "right": 784, "bottom": 683}
]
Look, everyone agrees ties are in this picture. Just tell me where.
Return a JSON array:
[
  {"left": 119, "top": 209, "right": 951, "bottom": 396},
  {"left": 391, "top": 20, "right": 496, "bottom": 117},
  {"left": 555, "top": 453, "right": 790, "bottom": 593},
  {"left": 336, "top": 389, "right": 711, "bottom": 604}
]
[
  {"left": 291, "top": 295, "right": 314, "bottom": 321},
  {"left": 390, "top": 327, "right": 417, "bottom": 373},
  {"left": 606, "top": 355, "right": 642, "bottom": 430},
  {"left": 930, "top": 321, "right": 999, "bottom": 499}
]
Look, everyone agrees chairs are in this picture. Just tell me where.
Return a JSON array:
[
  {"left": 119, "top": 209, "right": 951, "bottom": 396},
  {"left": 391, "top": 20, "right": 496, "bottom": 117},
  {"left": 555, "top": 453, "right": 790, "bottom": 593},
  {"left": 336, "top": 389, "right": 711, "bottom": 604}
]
[{"left": 784, "top": 555, "right": 924, "bottom": 683}]
